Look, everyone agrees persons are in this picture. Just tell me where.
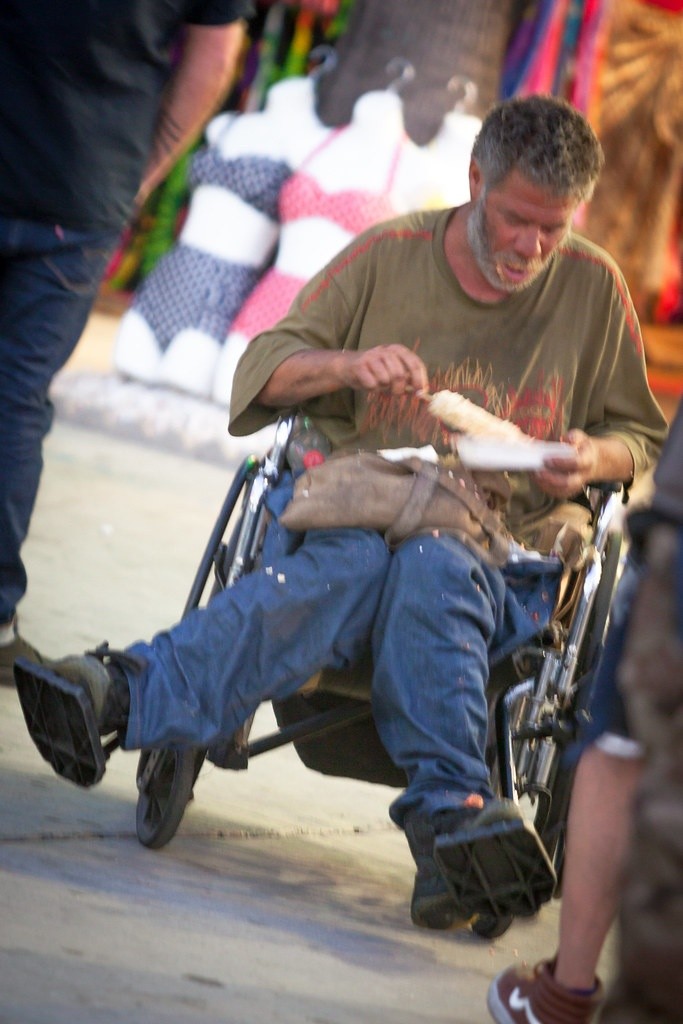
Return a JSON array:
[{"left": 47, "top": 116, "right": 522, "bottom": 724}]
[
  {"left": 49, "top": 88, "right": 673, "bottom": 934},
  {"left": 487, "top": 394, "right": 683, "bottom": 1024},
  {"left": 111, "top": 72, "right": 484, "bottom": 413},
  {"left": 0, "top": 0, "right": 257, "bottom": 682}
]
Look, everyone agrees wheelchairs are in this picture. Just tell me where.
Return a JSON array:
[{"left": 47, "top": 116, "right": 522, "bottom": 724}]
[{"left": 11, "top": 408, "right": 627, "bottom": 942}]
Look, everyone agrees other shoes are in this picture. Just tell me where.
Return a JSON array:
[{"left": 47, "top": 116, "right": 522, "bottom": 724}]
[
  {"left": 412, "top": 800, "right": 519, "bottom": 930},
  {"left": 0, "top": 619, "right": 50, "bottom": 684},
  {"left": 489, "top": 960, "right": 604, "bottom": 1024},
  {"left": 58, "top": 642, "right": 140, "bottom": 738}
]
